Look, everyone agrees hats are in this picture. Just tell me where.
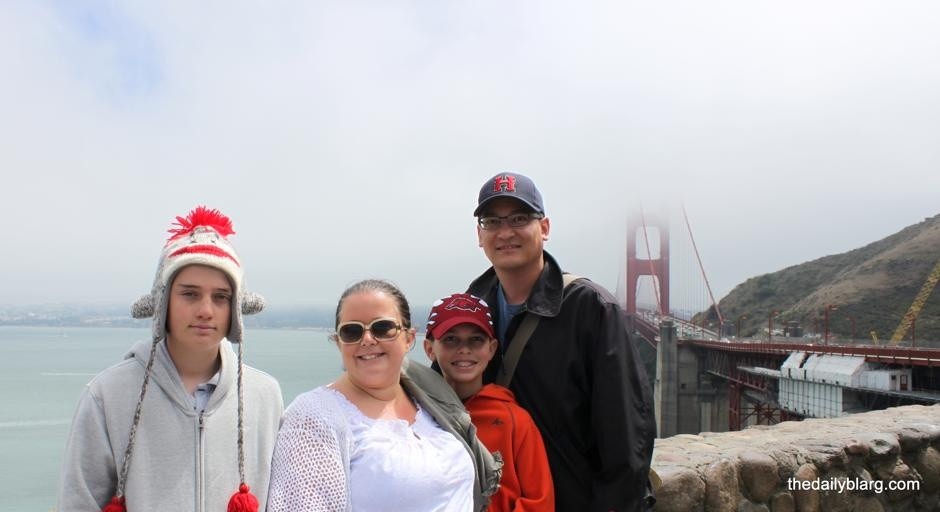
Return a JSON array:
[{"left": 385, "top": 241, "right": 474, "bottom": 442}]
[
  {"left": 425, "top": 294, "right": 495, "bottom": 340},
  {"left": 474, "top": 171, "right": 545, "bottom": 216},
  {"left": 101, "top": 206, "right": 265, "bottom": 512}
]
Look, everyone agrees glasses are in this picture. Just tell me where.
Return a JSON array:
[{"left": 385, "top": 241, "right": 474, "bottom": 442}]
[
  {"left": 336, "top": 318, "right": 407, "bottom": 345},
  {"left": 478, "top": 210, "right": 542, "bottom": 230}
]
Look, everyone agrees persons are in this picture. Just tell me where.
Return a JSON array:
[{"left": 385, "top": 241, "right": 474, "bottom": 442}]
[
  {"left": 57, "top": 224, "right": 285, "bottom": 512},
  {"left": 422, "top": 292, "right": 556, "bottom": 512},
  {"left": 265, "top": 278, "right": 479, "bottom": 512},
  {"left": 431, "top": 170, "right": 656, "bottom": 511}
]
